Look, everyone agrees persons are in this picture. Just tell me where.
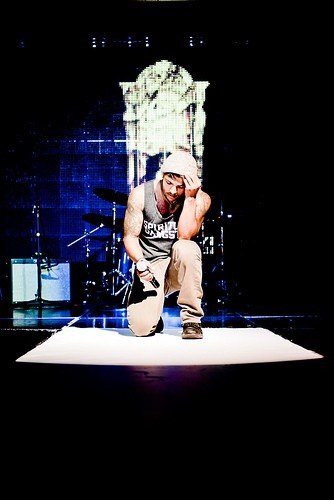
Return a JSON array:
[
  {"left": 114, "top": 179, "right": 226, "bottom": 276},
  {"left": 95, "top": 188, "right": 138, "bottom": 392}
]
[{"left": 123, "top": 151, "right": 211, "bottom": 339}]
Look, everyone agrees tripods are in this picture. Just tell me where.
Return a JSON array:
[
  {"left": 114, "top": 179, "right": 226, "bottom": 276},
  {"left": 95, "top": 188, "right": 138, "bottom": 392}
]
[{"left": 11, "top": 198, "right": 70, "bottom": 312}]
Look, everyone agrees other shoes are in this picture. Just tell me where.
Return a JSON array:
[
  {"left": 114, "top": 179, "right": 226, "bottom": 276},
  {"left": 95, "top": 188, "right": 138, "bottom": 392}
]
[
  {"left": 155, "top": 316, "right": 164, "bottom": 332},
  {"left": 181, "top": 323, "right": 203, "bottom": 339}
]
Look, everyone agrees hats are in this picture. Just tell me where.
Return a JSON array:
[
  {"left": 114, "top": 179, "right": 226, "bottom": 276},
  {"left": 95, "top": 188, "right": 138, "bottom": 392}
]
[{"left": 161, "top": 151, "right": 197, "bottom": 179}]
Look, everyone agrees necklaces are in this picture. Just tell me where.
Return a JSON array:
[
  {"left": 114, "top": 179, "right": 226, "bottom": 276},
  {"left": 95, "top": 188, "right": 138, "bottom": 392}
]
[{"left": 163, "top": 193, "right": 180, "bottom": 211}]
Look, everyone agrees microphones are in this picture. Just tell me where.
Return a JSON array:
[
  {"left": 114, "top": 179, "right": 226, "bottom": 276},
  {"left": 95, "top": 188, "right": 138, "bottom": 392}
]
[{"left": 136, "top": 259, "right": 160, "bottom": 289}]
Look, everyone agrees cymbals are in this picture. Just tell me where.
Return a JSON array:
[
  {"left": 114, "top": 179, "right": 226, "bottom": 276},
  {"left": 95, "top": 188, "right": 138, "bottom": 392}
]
[
  {"left": 93, "top": 187, "right": 128, "bottom": 206},
  {"left": 82, "top": 212, "right": 124, "bottom": 233}
]
